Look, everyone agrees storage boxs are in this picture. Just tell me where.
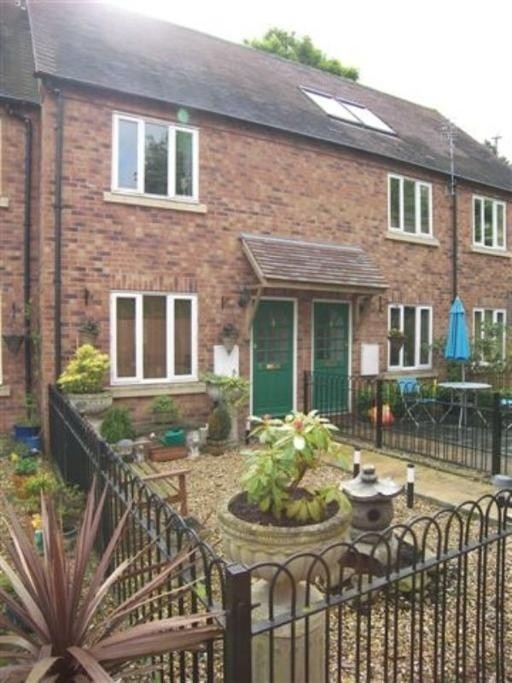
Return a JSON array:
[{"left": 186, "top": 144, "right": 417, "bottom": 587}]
[{"left": 148, "top": 441, "right": 194, "bottom": 464}]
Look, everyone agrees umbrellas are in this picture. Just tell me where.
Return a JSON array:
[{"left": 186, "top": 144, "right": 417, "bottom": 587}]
[{"left": 442, "top": 291, "right": 475, "bottom": 385}]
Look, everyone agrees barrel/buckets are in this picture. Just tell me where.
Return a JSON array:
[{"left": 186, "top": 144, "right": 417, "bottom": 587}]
[{"left": 13, "top": 422, "right": 43, "bottom": 457}]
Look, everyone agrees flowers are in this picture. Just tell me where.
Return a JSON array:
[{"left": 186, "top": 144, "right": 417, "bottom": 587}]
[{"left": 51, "top": 338, "right": 116, "bottom": 444}]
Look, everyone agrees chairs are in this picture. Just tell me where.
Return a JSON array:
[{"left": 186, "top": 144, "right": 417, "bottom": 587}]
[
  {"left": 502, "top": 397, "right": 512, "bottom": 431},
  {"left": 396, "top": 375, "right": 439, "bottom": 431}
]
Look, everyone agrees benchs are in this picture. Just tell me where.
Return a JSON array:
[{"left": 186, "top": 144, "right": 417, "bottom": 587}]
[{"left": 109, "top": 439, "right": 194, "bottom": 524}]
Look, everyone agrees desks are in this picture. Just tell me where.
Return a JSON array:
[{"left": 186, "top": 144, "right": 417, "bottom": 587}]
[{"left": 434, "top": 379, "right": 494, "bottom": 431}]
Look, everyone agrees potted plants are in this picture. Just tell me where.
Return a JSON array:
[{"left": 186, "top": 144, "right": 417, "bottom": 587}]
[
  {"left": 150, "top": 397, "right": 180, "bottom": 427},
  {"left": 54, "top": 484, "right": 89, "bottom": 559},
  {"left": 218, "top": 322, "right": 240, "bottom": 356},
  {"left": 211, "top": 404, "right": 350, "bottom": 644},
  {"left": 15, "top": 458, "right": 40, "bottom": 490},
  {"left": 77, "top": 317, "right": 103, "bottom": 347},
  {"left": 199, "top": 371, "right": 254, "bottom": 406},
  {"left": 387, "top": 327, "right": 410, "bottom": 356},
  {"left": 9, "top": 417, "right": 41, "bottom": 437}
]
